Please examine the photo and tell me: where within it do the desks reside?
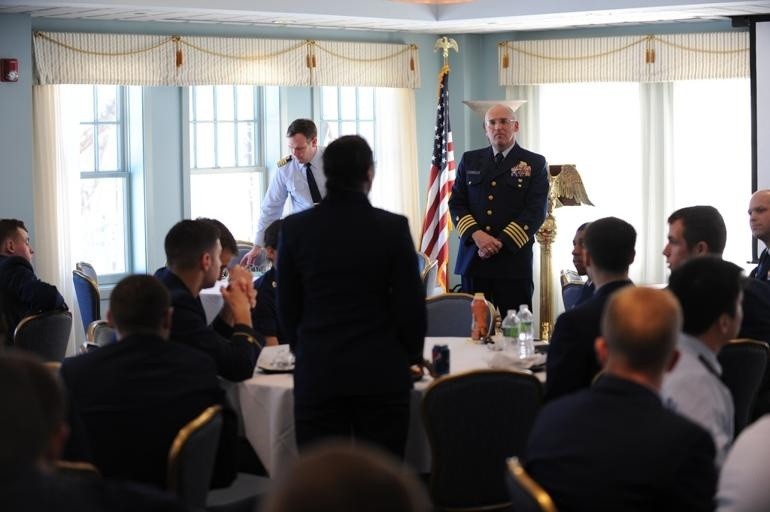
[238,336,554,474]
[193,271,261,324]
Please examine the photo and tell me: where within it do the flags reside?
[419,66,460,293]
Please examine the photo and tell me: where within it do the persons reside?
[448,103,551,314]
[0,189,770,512]
[239,119,328,271]
[273,134,426,460]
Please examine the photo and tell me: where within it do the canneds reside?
[432,344,449,378]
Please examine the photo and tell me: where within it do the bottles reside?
[471,292,535,362]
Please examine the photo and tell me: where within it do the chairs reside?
[500,450,556,512]
[422,369,542,510]
[425,292,495,338]
[98,404,229,511]
[71,264,116,347]
[14,311,72,365]
[715,335,768,433]
[561,282,588,312]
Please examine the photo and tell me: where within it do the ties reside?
[496,153,503,167]
[306,162,322,207]
